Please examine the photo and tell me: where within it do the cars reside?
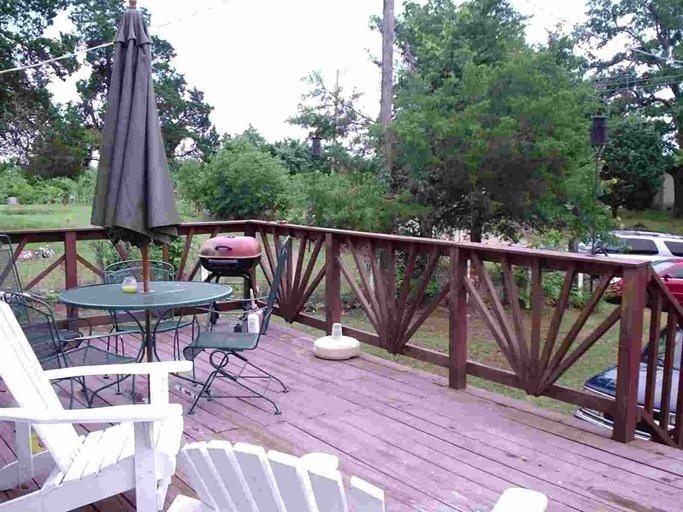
[574,325,681,444]
[566,229,681,312]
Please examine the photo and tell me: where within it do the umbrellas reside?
[89,2,180,292]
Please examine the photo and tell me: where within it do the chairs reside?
[165,437,549,512]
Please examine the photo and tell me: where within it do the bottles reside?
[121,275,138,293]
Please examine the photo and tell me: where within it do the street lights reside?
[309,128,321,222]
[588,105,609,297]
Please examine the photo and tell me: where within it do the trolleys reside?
[196,232,261,321]
